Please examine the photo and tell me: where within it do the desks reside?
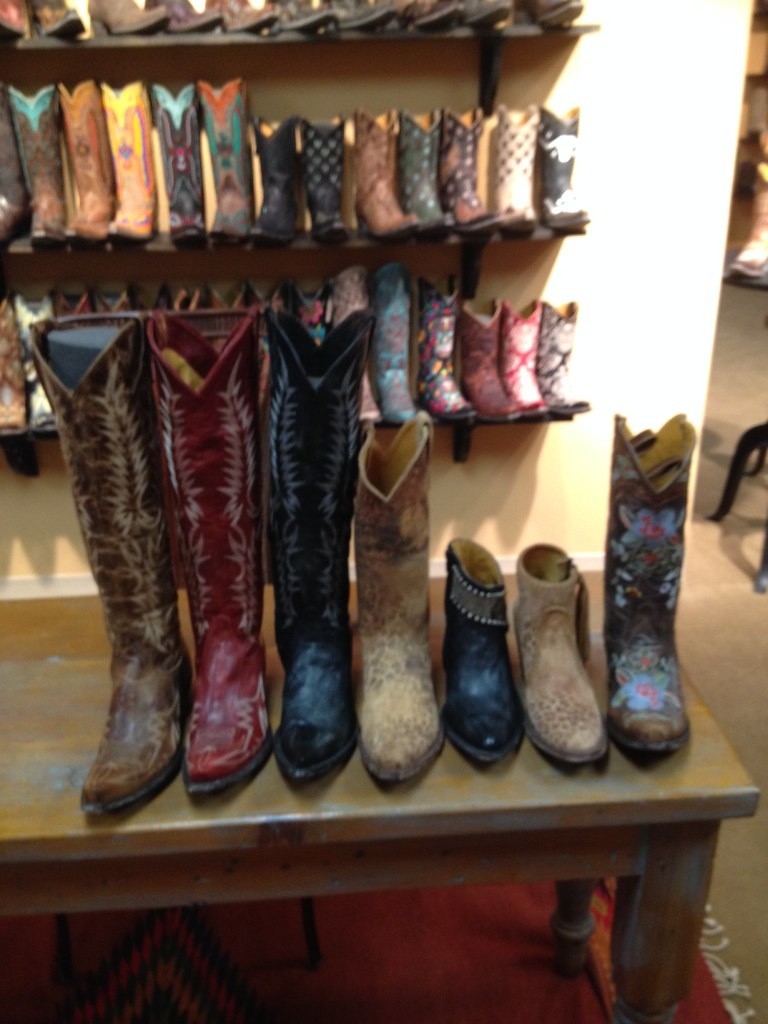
[0,574,760,1024]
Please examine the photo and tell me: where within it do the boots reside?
[0,262,590,440]
[440,538,523,762]
[9,84,68,246]
[0,82,28,245]
[604,414,697,754]
[150,83,206,245]
[0,0,584,45]
[354,107,419,238]
[495,104,537,232]
[266,305,358,790]
[249,116,295,243]
[57,80,112,244]
[352,412,441,784]
[398,108,456,234]
[197,78,254,241]
[442,108,502,233]
[100,81,158,243]
[540,104,590,228]
[146,307,271,796]
[28,311,191,816]
[299,116,347,236]
[515,544,606,763]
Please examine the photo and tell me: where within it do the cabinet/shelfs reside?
[0,0,601,479]
